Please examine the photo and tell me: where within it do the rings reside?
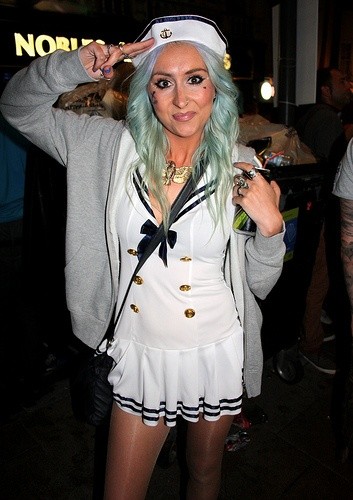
[108,44,115,56]
[236,174,249,189]
[237,186,243,195]
[120,43,124,54]
[245,165,258,180]
[123,53,128,59]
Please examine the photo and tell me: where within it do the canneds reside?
[263,151,293,166]
[232,166,270,236]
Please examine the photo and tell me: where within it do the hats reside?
[128,15,229,71]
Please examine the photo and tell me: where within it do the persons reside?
[0,14,286,500]
[294,66,353,374]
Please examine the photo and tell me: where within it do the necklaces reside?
[161,160,193,185]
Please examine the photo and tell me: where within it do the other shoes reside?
[324,332,335,342]
[320,310,331,325]
[304,345,337,376]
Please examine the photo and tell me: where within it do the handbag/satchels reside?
[88,354,114,425]
